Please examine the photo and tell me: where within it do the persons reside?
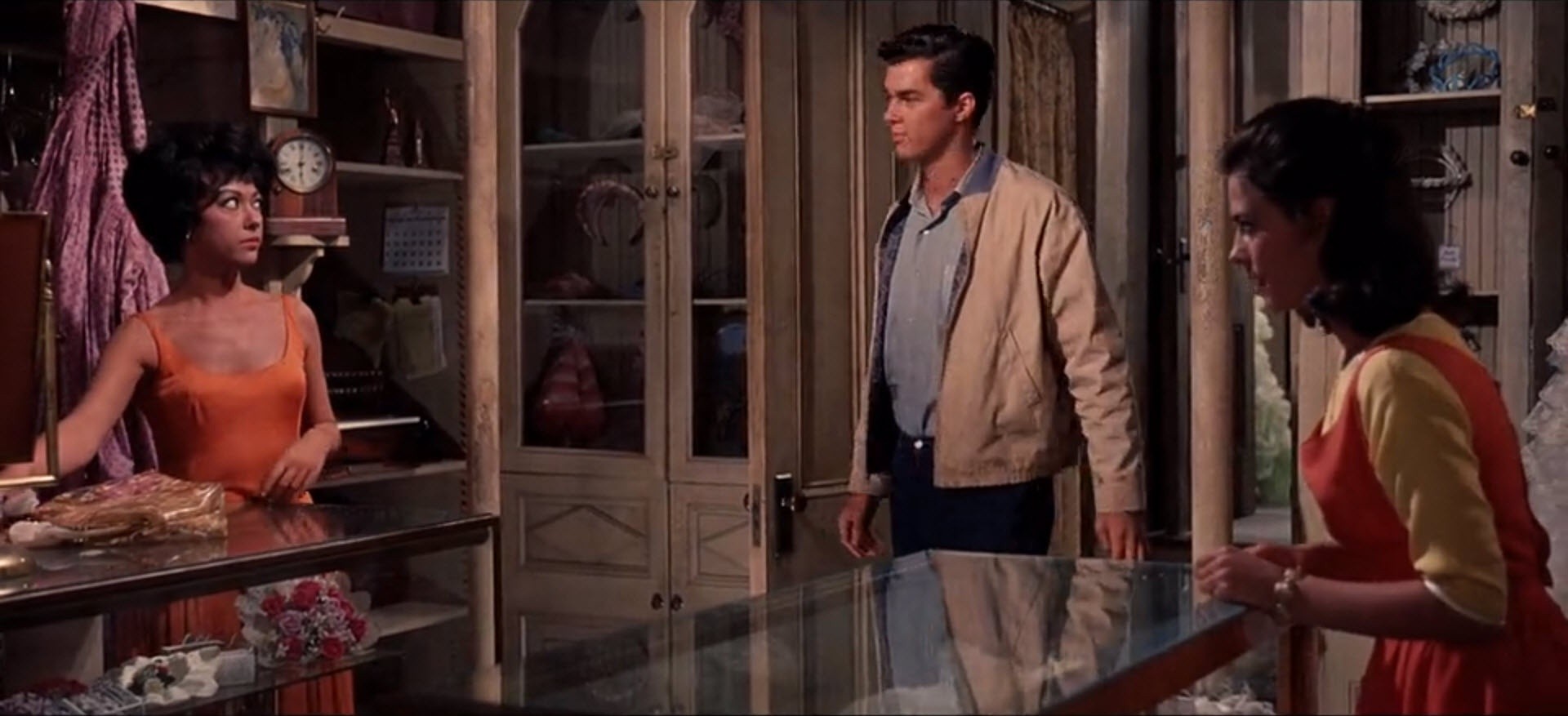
[1192,96,1568,716]
[837,21,1147,560]
[0,118,355,716]
[869,550,1134,716]
[522,214,606,447]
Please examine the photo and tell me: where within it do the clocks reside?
[263,127,347,238]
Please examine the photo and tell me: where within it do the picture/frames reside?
[242,0,319,119]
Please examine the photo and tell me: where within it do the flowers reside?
[233,571,374,666]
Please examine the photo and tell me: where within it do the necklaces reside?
[1328,367,1338,426]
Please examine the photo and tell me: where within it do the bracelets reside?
[1274,566,1309,615]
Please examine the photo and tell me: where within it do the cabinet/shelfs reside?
[309,13,466,641]
[1303,1,1568,716]
[497,0,800,662]
